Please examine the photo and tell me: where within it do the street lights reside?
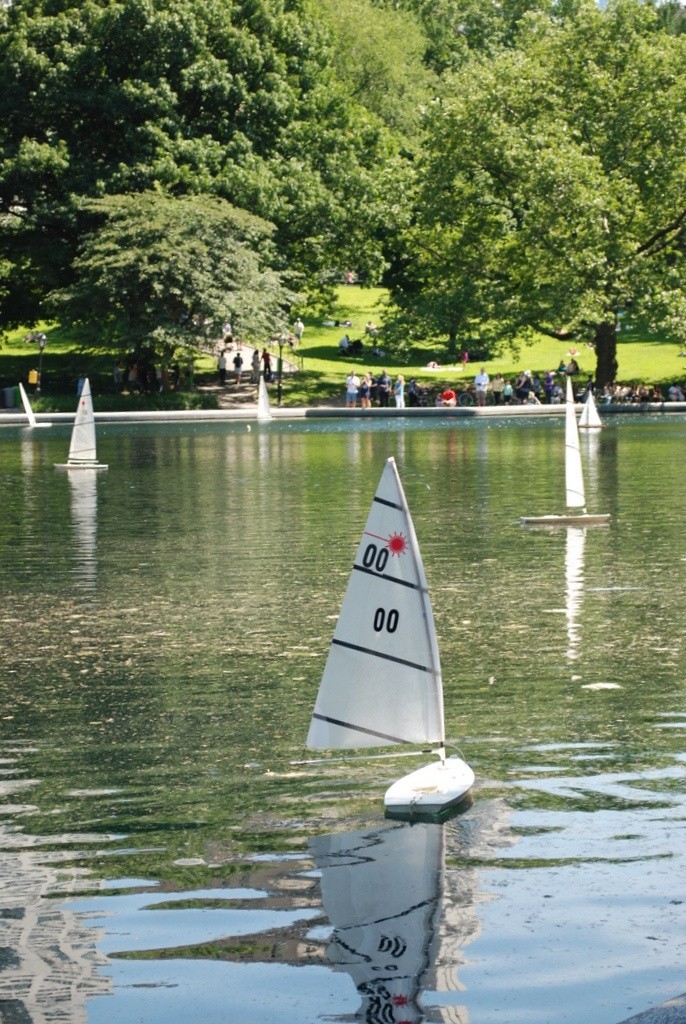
[276,334,287,407]
[33,334,49,402]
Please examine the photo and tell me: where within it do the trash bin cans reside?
[11,386,21,407]
[0,387,16,408]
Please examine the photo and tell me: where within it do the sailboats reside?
[253,375,277,421]
[18,382,54,429]
[303,453,477,822]
[576,389,608,429]
[518,374,614,526]
[304,813,471,1023]
[53,376,110,470]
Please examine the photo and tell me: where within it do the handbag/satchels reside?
[265,373,274,382]
[252,361,259,368]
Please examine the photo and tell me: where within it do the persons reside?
[23,270,686,407]
